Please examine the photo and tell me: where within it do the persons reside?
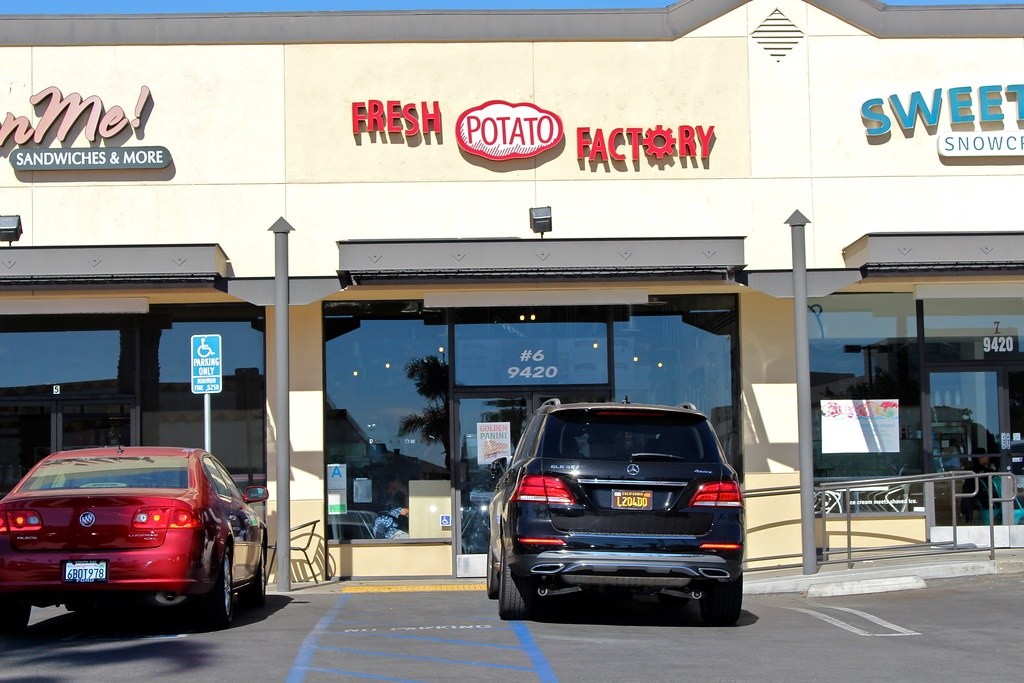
[372,471,408,539]
[960,447,1001,526]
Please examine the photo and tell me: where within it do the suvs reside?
[487,399,748,628]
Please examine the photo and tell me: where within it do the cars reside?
[0,447,269,639]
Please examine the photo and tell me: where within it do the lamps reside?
[529,205,552,238]
[0,214,23,246]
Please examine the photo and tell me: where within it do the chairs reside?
[972,477,1024,525]
[268,519,320,584]
[332,510,378,540]
